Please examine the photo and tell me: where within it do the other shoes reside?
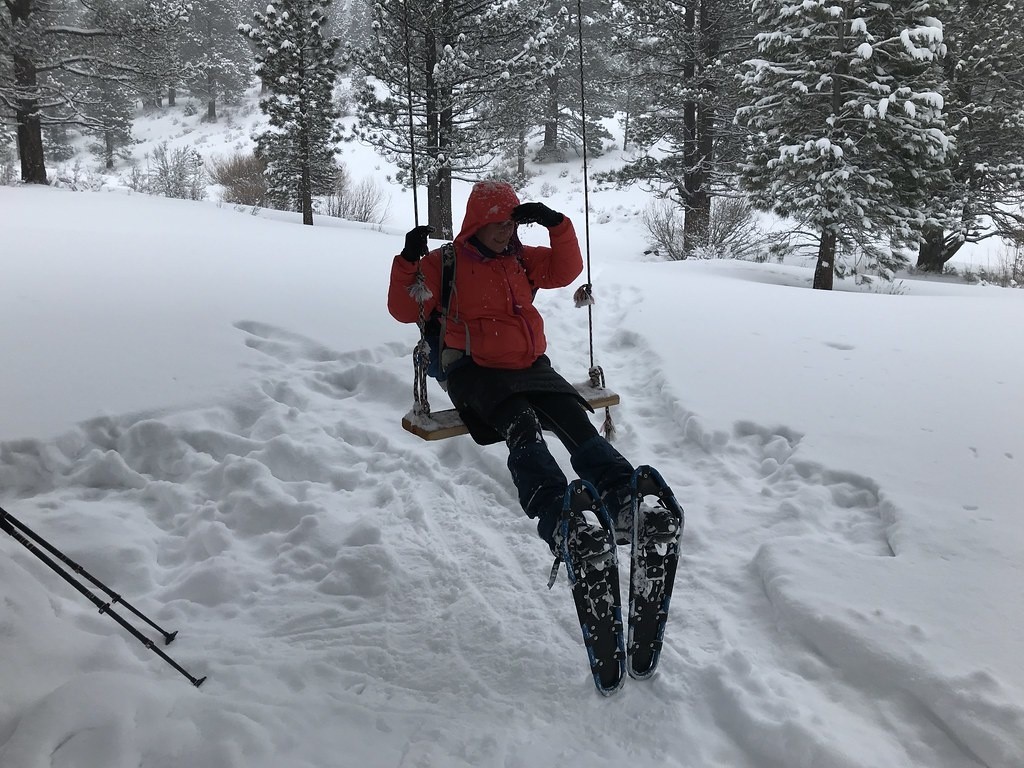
[615,495,678,545]
[551,522,608,561]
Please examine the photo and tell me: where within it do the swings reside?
[396,1,620,442]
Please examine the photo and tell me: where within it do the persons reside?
[385,178,672,567]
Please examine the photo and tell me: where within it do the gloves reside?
[400,225,437,265]
[511,202,563,228]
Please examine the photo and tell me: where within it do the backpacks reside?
[424,241,456,382]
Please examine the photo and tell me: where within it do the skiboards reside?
[561,463,681,695]
